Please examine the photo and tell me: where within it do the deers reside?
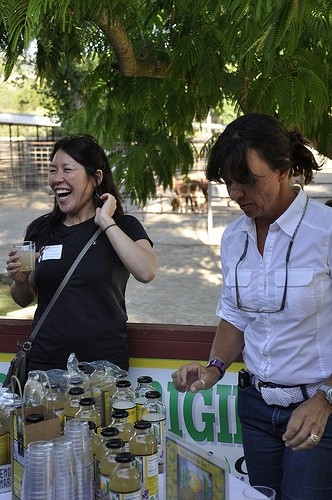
[171,178,209,214]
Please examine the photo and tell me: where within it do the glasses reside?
[63,133,99,153]
[235,240,293,314]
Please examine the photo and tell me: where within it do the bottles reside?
[23,370,43,409]
[0,388,22,466]
[42,383,65,436]
[25,414,44,425]
[65,363,167,500]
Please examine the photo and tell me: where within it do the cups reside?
[243,485,276,500]
[12,240,36,272]
[20,419,96,499]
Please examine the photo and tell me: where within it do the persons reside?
[6,133,156,396]
[172,112,332,500]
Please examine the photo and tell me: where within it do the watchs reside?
[317,384,332,405]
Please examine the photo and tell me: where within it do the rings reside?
[308,434,319,444]
[200,380,205,390]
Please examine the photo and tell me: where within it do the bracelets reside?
[205,358,225,381]
[104,224,117,233]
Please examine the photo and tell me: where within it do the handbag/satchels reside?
[2,351,27,397]
